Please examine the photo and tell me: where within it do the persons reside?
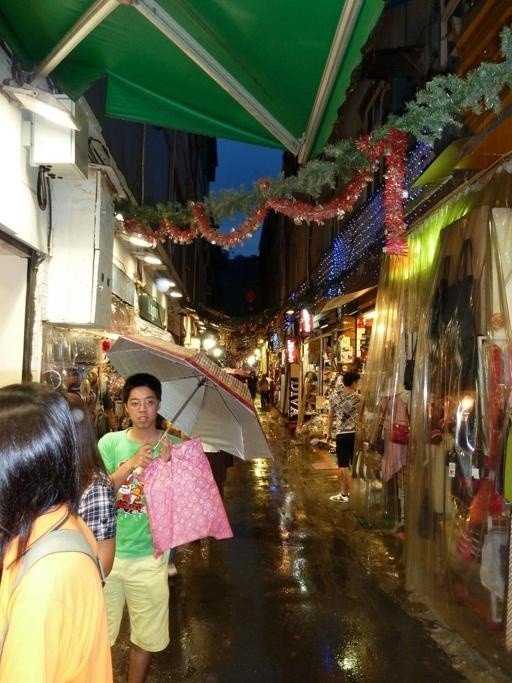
[0,382,114,683]
[249,371,273,412]
[96,373,188,683]
[325,371,362,503]
[62,392,116,578]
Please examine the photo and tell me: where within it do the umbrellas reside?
[107,334,274,465]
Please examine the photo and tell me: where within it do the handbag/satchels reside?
[480,529,509,600]
[403,358,415,390]
[445,275,474,325]
[366,434,386,456]
[133,436,233,559]
[390,424,411,445]
[352,451,382,481]
[354,428,369,450]
[429,286,443,339]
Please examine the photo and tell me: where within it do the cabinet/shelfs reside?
[300,316,355,447]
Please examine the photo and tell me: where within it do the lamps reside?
[130,251,162,264]
[115,230,152,248]
[342,305,359,315]
[2,84,82,132]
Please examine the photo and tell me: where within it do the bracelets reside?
[327,435,331,437]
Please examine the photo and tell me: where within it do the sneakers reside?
[168,561,177,576]
[329,492,350,502]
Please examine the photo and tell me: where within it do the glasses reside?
[127,399,161,409]
